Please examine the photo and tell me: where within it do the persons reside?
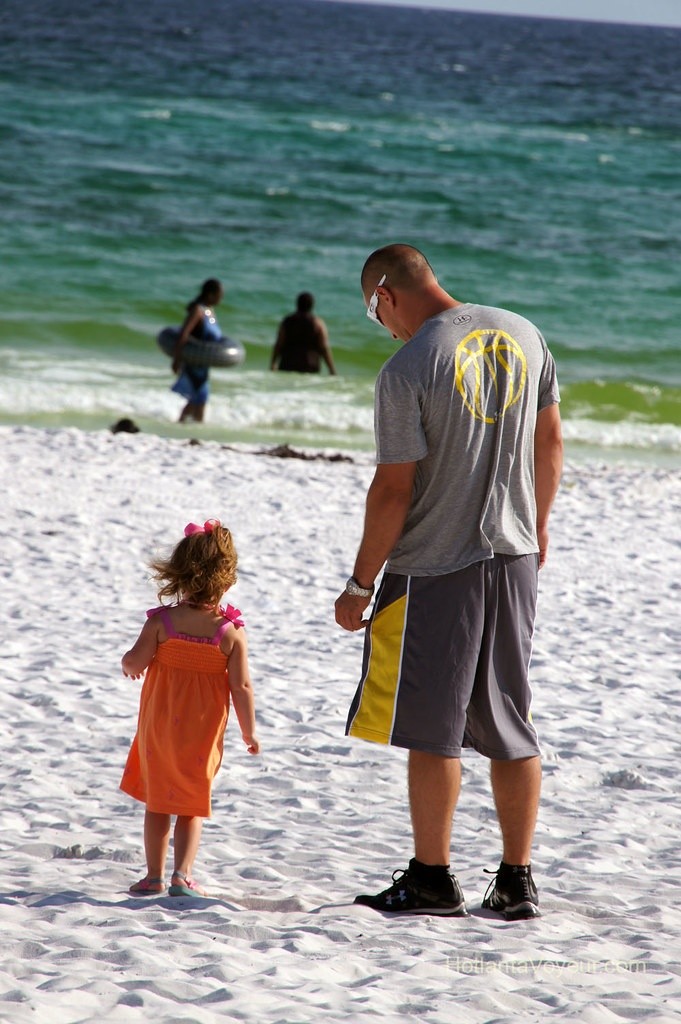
[172,277,226,425]
[334,244,565,922]
[268,291,336,376]
[120,520,260,897]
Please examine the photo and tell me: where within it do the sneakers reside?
[484,860,543,920]
[353,858,469,917]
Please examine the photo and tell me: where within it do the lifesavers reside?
[155,327,246,368]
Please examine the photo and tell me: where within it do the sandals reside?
[168,872,193,895]
[129,877,163,894]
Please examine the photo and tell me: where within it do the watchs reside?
[345,577,375,598]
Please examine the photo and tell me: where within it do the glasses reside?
[365,274,387,331]
